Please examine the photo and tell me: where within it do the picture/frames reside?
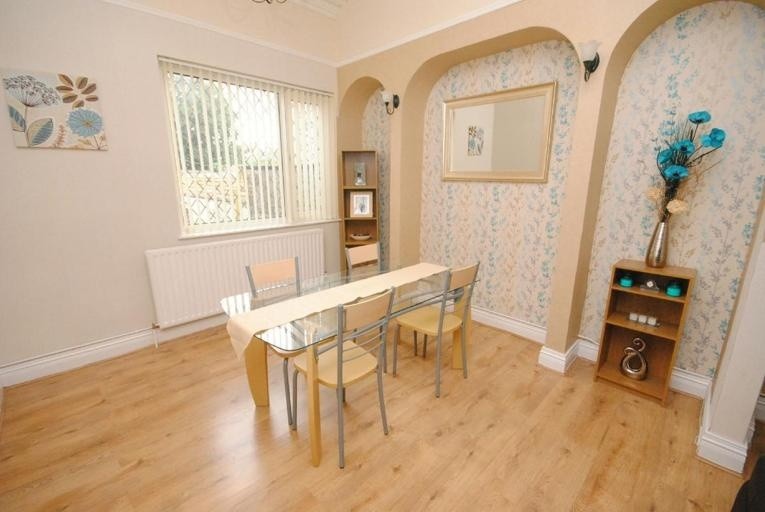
[348,189,375,221]
[437,81,559,185]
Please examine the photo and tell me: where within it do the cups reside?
[628,311,658,328]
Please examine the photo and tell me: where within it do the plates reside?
[349,234,372,240]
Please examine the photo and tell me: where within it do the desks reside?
[220,256,470,465]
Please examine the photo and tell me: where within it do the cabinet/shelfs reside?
[591,259,698,407]
[337,148,384,277]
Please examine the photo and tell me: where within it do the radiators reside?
[143,227,326,331]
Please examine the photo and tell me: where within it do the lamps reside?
[579,39,602,79]
[382,91,399,116]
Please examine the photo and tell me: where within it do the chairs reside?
[345,238,414,355]
[291,281,399,471]
[245,257,333,430]
[393,259,482,398]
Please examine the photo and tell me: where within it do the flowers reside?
[647,106,731,223]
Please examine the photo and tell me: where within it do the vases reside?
[647,216,668,267]
